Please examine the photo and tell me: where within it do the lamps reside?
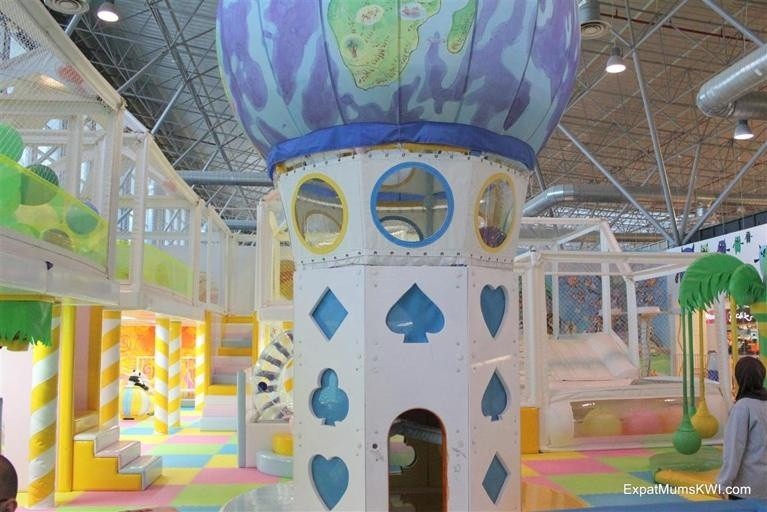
[604,47,627,74]
[96,0,119,22]
[733,119,754,140]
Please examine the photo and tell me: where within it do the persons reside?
[713,356,767,500]
[706,349,719,382]
[751,333,759,342]
[0,454,19,512]
[726,330,732,354]
[747,338,759,355]
[741,340,751,352]
